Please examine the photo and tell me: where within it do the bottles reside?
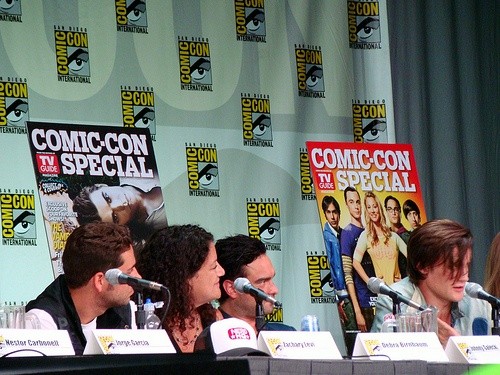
[145,299,162,329]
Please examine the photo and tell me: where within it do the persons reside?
[371,219,492,349]
[71,179,169,229]
[215,233,298,356]
[22,223,144,355]
[136,224,223,354]
[321,186,423,332]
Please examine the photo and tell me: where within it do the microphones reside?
[105,268,168,291]
[367,276,425,311]
[465,282,500,307]
[233,277,280,306]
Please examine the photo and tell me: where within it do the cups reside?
[301,315,320,332]
[396,308,438,336]
[3,306,40,329]
